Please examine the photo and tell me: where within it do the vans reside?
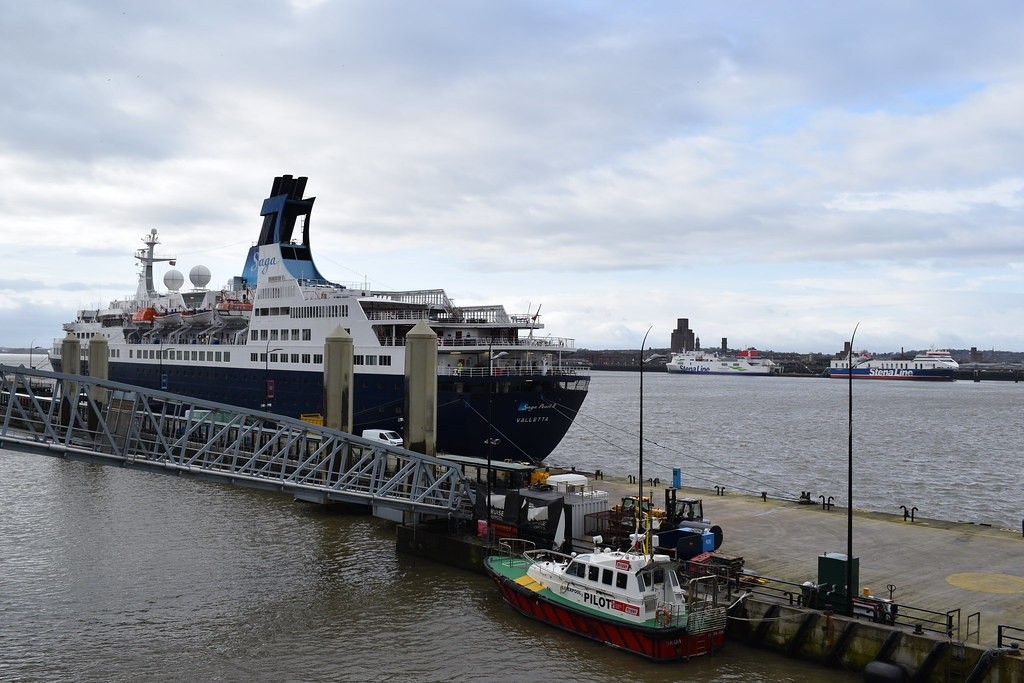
[361,429,404,448]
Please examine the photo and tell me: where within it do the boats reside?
[131,307,158,325]
[153,311,182,328]
[214,288,254,322]
[480,488,726,661]
[0,342,53,397]
[180,309,214,326]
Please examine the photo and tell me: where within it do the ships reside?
[46,174,592,467]
[827,347,960,382]
[666,346,785,375]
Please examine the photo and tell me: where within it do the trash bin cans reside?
[673,467,682,488]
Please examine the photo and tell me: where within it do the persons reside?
[505,361,510,369]
[515,361,521,375]
[532,362,537,369]
[457,360,463,372]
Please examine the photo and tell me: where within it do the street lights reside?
[639,323,668,533]
[484,344,508,543]
[260,332,283,412]
[847,322,878,620]
[159,338,176,391]
[29,339,42,389]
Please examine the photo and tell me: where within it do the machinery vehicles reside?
[612,492,667,520]
[659,486,704,532]
